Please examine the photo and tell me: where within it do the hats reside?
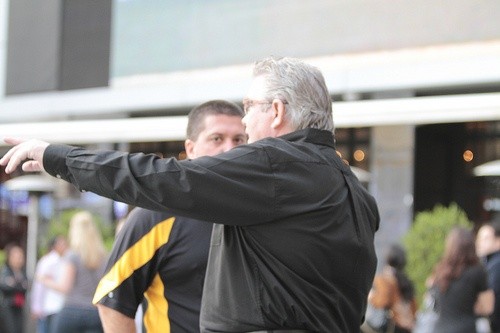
[448,226,471,245]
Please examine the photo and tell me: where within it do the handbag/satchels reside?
[367,311,386,331]
[412,299,438,333]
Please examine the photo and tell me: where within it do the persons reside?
[372,241,416,333]
[95,98,249,332]
[0,56,380,332]
[4,211,129,332]
[473,219,500,302]
[424,227,494,332]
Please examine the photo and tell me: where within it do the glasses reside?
[242,98,272,112]
